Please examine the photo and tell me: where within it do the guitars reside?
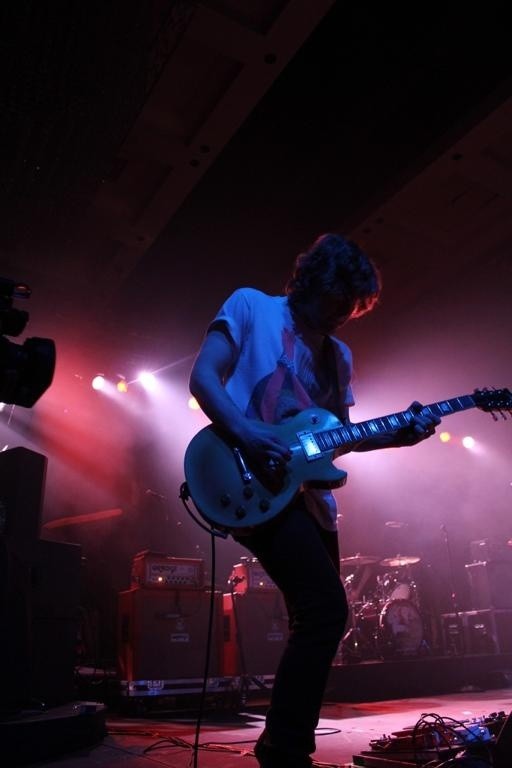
[183,388,512,535]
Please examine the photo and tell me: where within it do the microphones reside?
[440,525,448,535]
[145,489,168,501]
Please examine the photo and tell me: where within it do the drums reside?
[355,602,381,634]
[346,601,364,628]
[376,573,413,602]
[362,598,425,659]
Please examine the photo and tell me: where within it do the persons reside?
[189,234,442,767]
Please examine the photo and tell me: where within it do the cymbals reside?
[340,554,381,565]
[380,556,422,567]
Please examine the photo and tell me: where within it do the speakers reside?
[116,588,223,681]
[223,592,293,676]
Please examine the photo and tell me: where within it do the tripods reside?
[332,563,385,663]
